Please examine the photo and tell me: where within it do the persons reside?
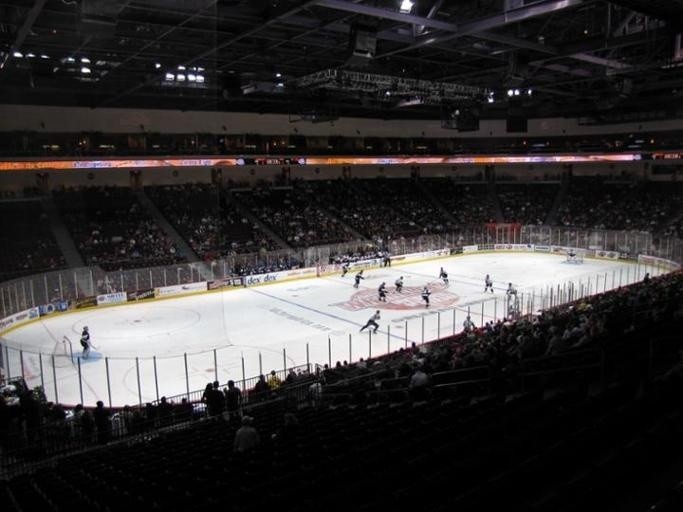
[334,307,560,364]
[352,268,365,288]
[0,365,681,510]
[247,174,559,278]
[482,273,494,294]
[376,281,388,303]
[393,275,404,293]
[560,174,682,365]
[505,282,518,298]
[0,181,248,318]
[436,266,449,288]
[79,325,93,359]
[420,285,431,307]
